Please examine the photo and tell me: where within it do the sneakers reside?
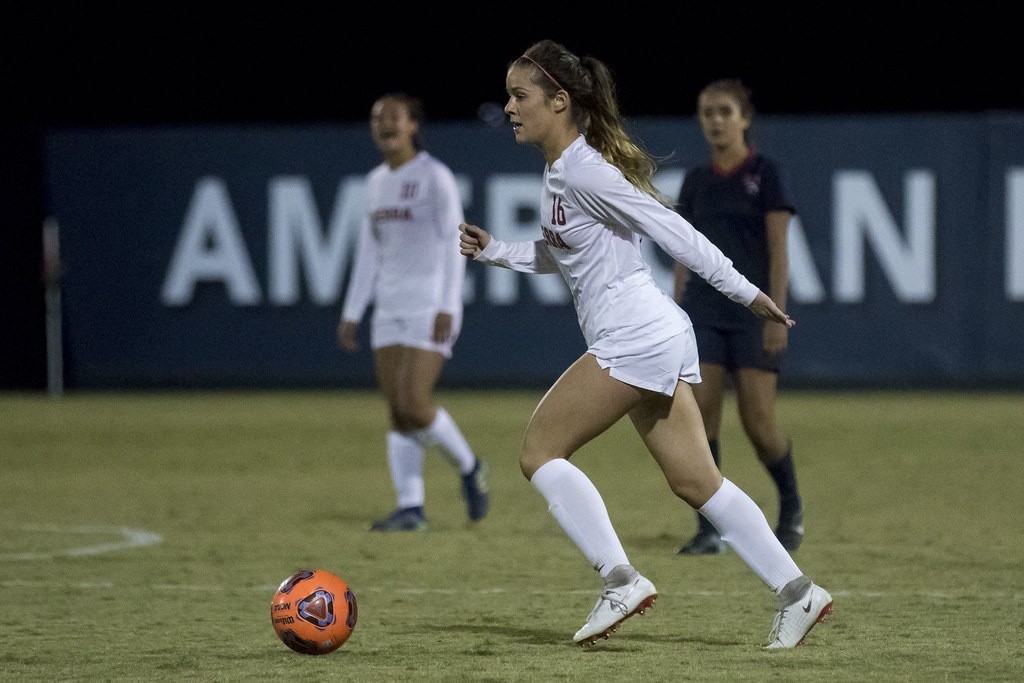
[573,573,658,647]
[775,501,806,550]
[370,506,429,533]
[763,580,834,649]
[460,455,491,521]
[678,526,722,555]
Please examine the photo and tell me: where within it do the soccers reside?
[269,568,359,656]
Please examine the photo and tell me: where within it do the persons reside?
[673,78,805,558]
[458,38,834,651]
[339,95,489,533]
